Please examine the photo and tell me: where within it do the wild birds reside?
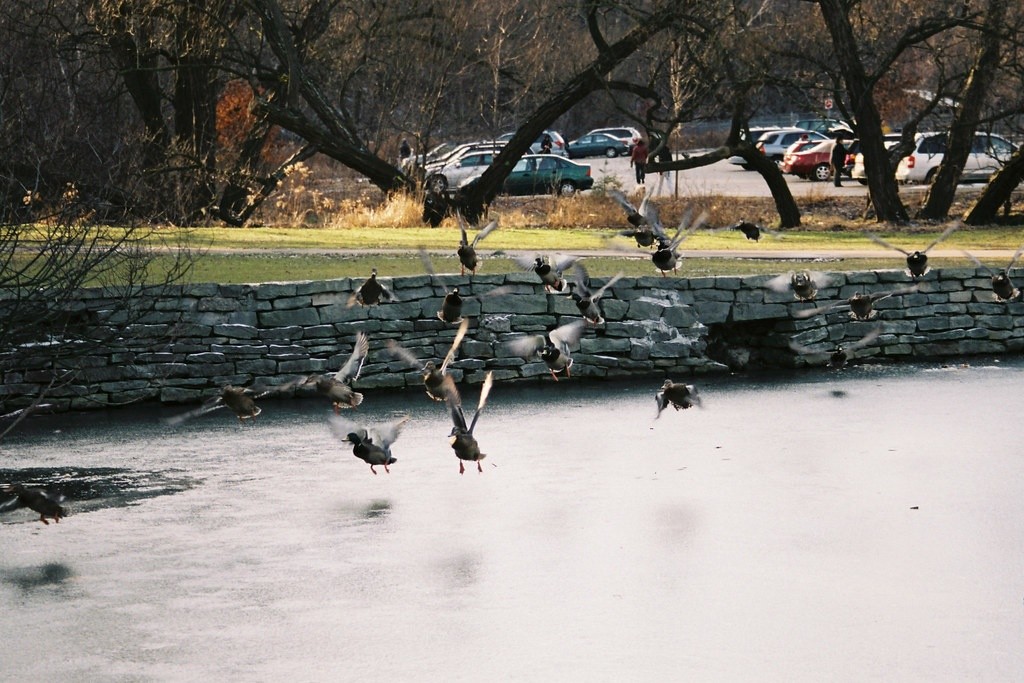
[0,480,73,525]
[963,247,1024,302]
[159,168,962,475]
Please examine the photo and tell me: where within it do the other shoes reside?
[835,185,844,187]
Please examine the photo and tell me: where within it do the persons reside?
[881,119,892,136]
[631,140,648,184]
[559,130,570,150]
[831,138,848,186]
[399,137,410,160]
[756,141,766,155]
[541,135,553,154]
[799,134,809,141]
[648,135,659,151]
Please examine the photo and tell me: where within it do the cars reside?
[498,153,594,198]
[893,129,1021,186]
[398,126,566,199]
[566,132,628,158]
[739,118,902,185]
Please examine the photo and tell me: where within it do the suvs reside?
[567,126,644,156]
